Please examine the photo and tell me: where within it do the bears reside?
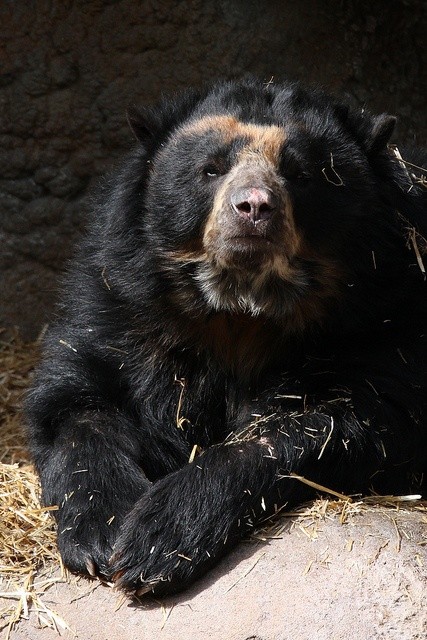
[21,72,427,600]
